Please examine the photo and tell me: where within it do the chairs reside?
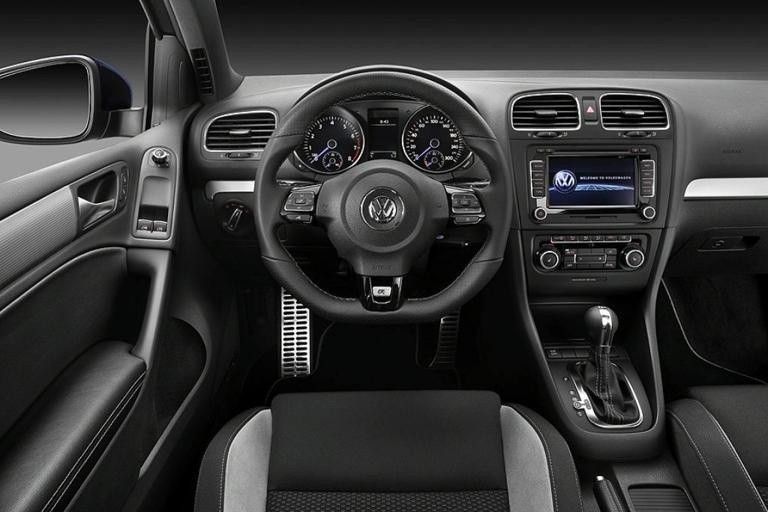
[668,385,767,511]
[191,384,581,511]
